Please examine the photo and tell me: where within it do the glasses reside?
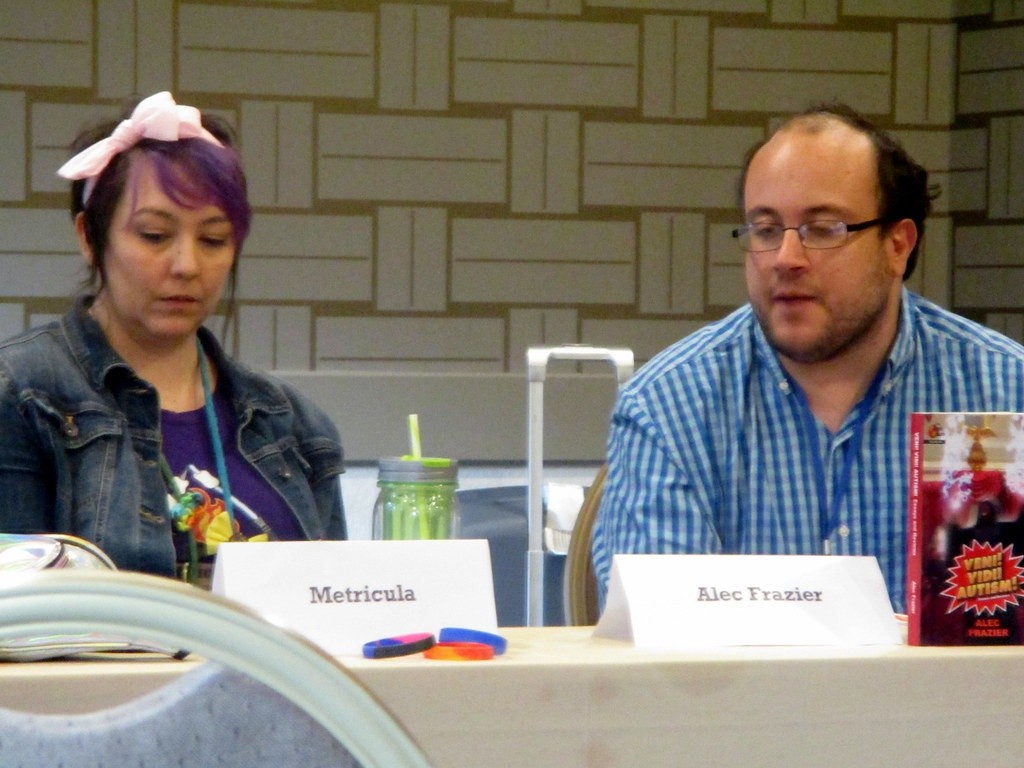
[732,218,893,253]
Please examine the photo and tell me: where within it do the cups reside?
[371,454,460,540]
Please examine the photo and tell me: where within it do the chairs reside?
[460,486,567,626]
[0,569,437,768]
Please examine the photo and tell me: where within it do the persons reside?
[592,102,1024,618]
[0,88,349,590]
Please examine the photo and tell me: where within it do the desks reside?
[0,625,1024,768]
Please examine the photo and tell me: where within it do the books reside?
[907,411,1024,647]
